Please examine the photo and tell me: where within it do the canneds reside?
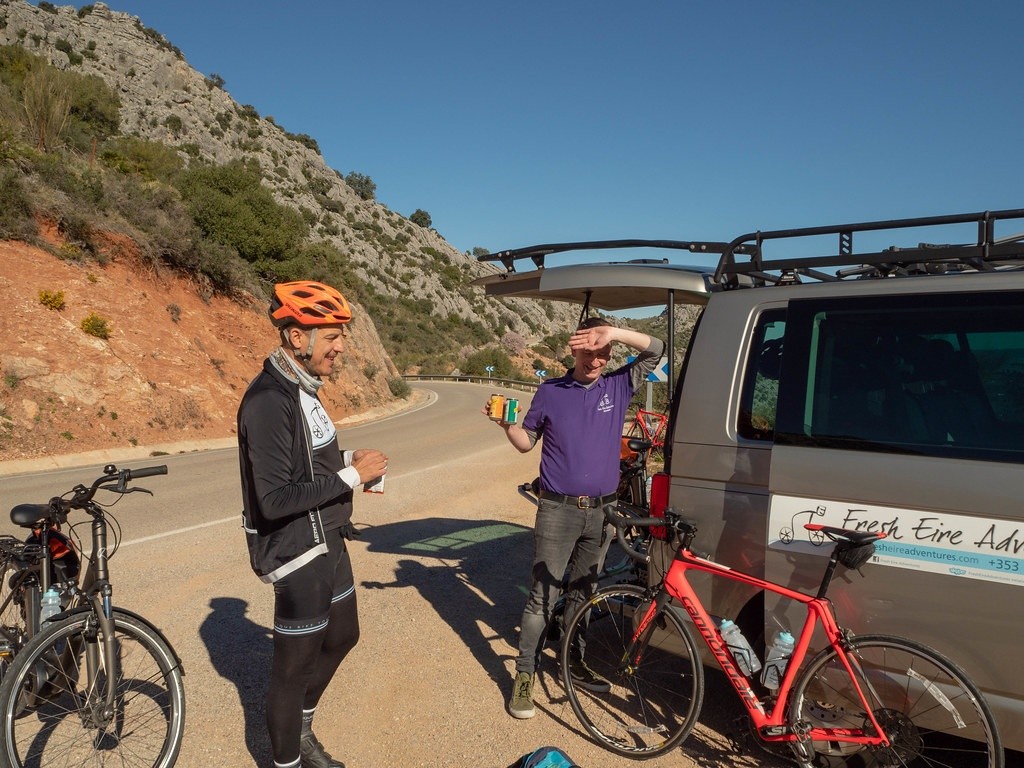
[489,393,504,420]
[504,397,518,425]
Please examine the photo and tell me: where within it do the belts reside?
[538,489,618,510]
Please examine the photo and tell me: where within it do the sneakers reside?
[508,671,536,718]
[558,660,612,692]
[300,733,345,768]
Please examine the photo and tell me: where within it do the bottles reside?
[760,629,795,689]
[645,476,652,503]
[39,588,61,631]
[719,618,762,676]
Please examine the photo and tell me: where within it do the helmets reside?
[268,280,352,328]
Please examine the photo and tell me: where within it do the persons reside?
[236,282,387,768]
[480,318,666,720]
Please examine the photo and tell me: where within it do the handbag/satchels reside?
[506,746,583,768]
[25,526,81,586]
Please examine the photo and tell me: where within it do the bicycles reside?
[561,500,1004,768]
[0,464,187,768]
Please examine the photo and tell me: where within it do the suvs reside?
[467,209,1024,755]
